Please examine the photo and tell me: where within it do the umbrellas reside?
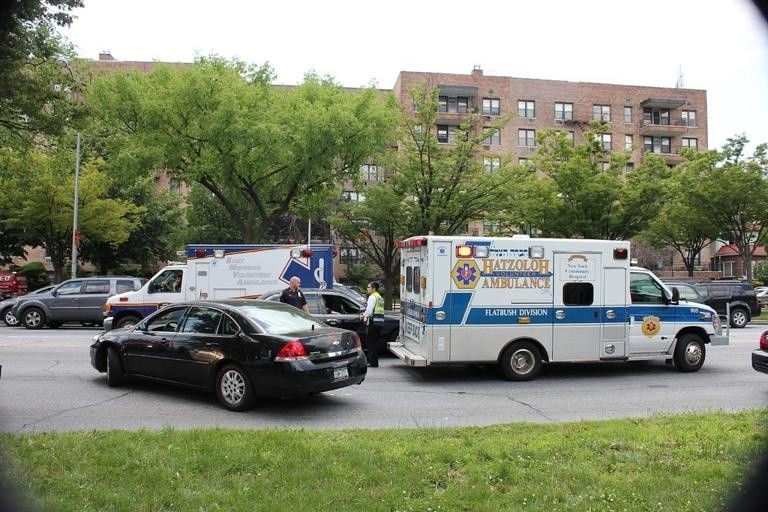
[366,363,379,367]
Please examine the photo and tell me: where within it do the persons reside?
[326,301,344,314]
[162,274,182,292]
[360,281,385,367]
[280,276,310,315]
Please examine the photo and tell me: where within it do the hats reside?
[635,275,768,375]
[258,287,402,357]
[333,282,367,306]
[0,285,96,330]
[86,298,370,414]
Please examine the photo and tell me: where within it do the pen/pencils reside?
[9,275,150,329]
